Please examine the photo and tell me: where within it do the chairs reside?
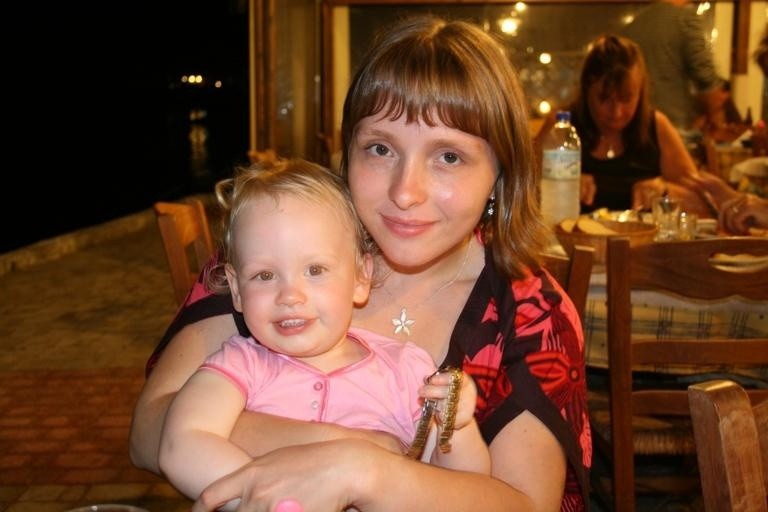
[153,199,216,304]
[516,123,768,512]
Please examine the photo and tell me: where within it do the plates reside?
[580,210,670,225]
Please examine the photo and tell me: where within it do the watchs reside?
[406,364,463,462]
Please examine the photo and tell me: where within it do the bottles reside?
[539,109,582,223]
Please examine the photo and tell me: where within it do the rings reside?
[729,206,739,216]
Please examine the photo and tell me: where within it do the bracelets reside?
[662,181,669,198]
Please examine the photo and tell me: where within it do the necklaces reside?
[370,227,473,336]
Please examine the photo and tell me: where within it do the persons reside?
[126,12,594,512]
[156,157,492,512]
[622,0,768,172]
[681,171,768,235]
[530,35,714,221]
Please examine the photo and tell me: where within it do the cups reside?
[652,196,682,240]
[679,212,699,240]
